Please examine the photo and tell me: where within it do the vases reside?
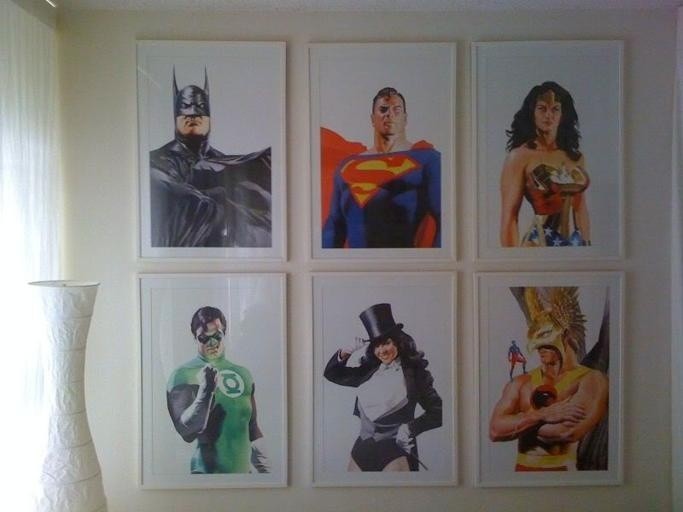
[27,275,113,511]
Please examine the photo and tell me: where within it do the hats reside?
[359,303,404,341]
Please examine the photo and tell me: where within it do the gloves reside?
[344,335,366,357]
[396,421,415,456]
[183,365,220,433]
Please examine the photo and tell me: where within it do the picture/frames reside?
[466,266,628,491]
[129,36,291,265]
[302,268,468,489]
[130,268,292,493]
[464,34,633,266]
[300,40,461,265]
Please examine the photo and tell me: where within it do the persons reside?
[166,305,275,473]
[494,80,593,247]
[488,310,609,472]
[321,299,442,472]
[149,61,275,251]
[505,340,529,381]
[321,87,442,249]
